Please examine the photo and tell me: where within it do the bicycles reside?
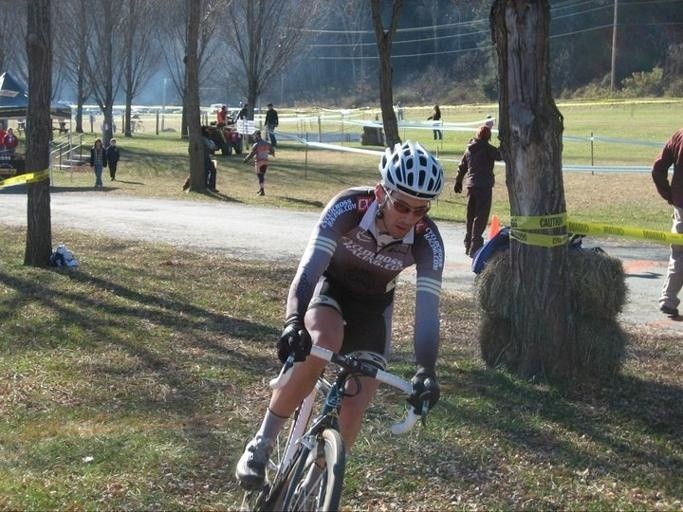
[130,120,145,133]
[238,342,429,512]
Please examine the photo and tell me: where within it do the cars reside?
[58,100,208,114]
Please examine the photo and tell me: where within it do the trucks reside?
[209,103,258,113]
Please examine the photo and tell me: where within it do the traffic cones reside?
[487,214,499,240]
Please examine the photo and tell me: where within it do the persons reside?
[2,128,18,152]
[397,101,404,121]
[236,140,445,512]
[485,116,494,141]
[131,112,141,133]
[90,140,107,188]
[427,105,442,140]
[201,103,278,196]
[652,129,683,314]
[106,138,120,181]
[0,123,6,152]
[454,126,504,258]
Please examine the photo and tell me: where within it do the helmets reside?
[378,139,443,201]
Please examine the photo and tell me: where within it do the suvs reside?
[201,119,245,155]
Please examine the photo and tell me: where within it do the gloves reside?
[277,313,312,363]
[454,182,462,193]
[406,359,440,415]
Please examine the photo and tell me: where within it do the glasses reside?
[380,185,431,216]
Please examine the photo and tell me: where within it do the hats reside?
[480,127,491,140]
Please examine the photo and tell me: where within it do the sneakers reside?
[661,306,678,314]
[235,436,273,489]
